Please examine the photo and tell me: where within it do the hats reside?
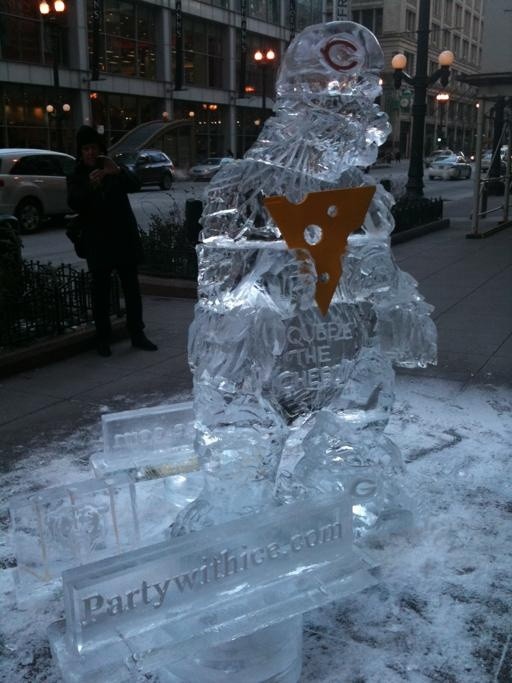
[74,123,110,162]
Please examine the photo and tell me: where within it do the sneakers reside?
[93,332,113,360]
[130,330,160,352]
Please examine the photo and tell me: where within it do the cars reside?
[426,141,512,183]
[188,156,236,182]
[432,90,450,148]
[0,146,84,230]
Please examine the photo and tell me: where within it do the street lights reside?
[254,45,278,137]
[390,52,455,201]
[39,0,71,150]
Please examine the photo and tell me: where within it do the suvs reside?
[107,149,179,194]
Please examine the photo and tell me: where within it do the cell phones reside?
[95,157,105,170]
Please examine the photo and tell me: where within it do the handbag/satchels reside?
[63,212,98,262]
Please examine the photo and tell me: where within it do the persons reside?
[385,151,392,163]
[64,124,158,358]
[211,151,218,164]
[459,148,466,160]
[380,149,385,161]
[226,150,233,157]
[395,151,401,164]
